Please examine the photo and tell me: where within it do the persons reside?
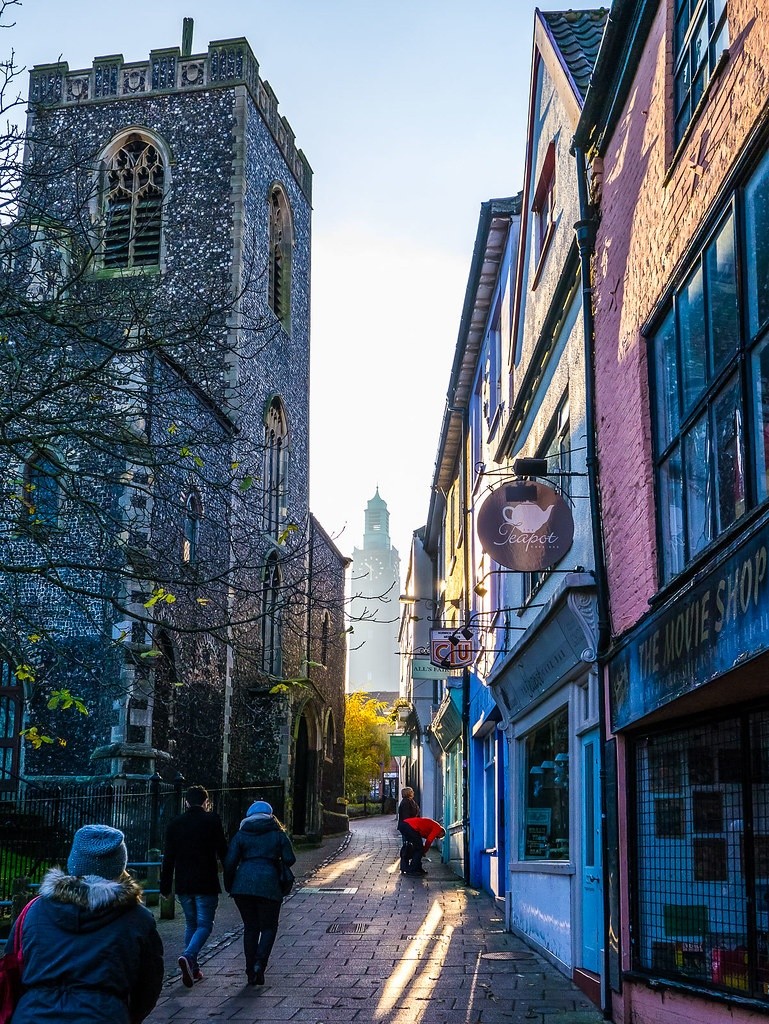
[397,787,421,876]
[225,801,297,987]
[398,817,446,875]
[160,787,228,988]
[0,825,165,1024]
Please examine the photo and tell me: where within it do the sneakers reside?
[178,956,204,988]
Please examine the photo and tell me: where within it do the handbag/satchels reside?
[400,842,414,860]
[1,895,45,1023]
[279,856,294,896]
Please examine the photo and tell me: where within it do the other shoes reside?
[411,862,428,875]
[245,964,266,986]
[401,865,415,874]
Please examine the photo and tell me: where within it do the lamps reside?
[449,625,526,646]
[473,569,575,598]
[530,751,569,797]
[510,458,548,477]
[462,603,544,641]
[440,649,509,667]
[399,595,459,609]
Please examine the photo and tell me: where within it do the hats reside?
[247,801,273,818]
[67,824,128,882]
